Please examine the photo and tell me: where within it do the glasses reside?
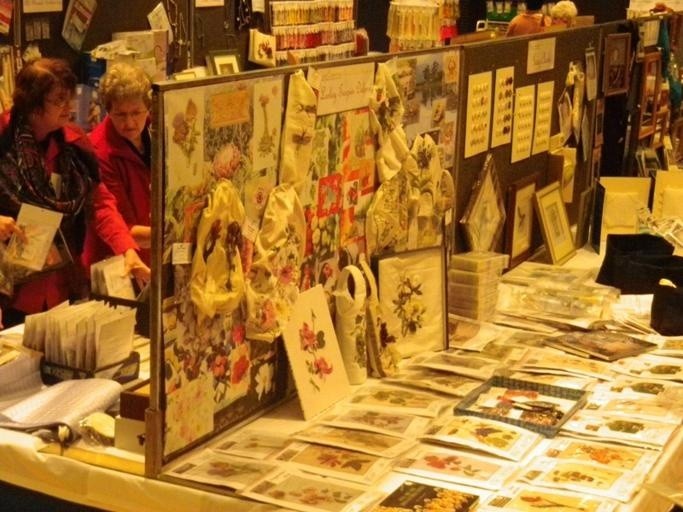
[112,108,149,120]
[46,97,66,108]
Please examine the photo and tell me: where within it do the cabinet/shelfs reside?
[636,47,658,154]
[655,106,673,151]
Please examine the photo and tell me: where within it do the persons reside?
[79,62,154,281]
[0,56,154,312]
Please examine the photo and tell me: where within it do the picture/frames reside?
[501,174,540,272]
[374,247,447,359]
[209,48,247,77]
[530,181,575,263]
[277,286,350,420]
[599,32,630,95]
[575,189,592,249]
[459,153,509,256]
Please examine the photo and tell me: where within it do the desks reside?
[1,14,681,512]
